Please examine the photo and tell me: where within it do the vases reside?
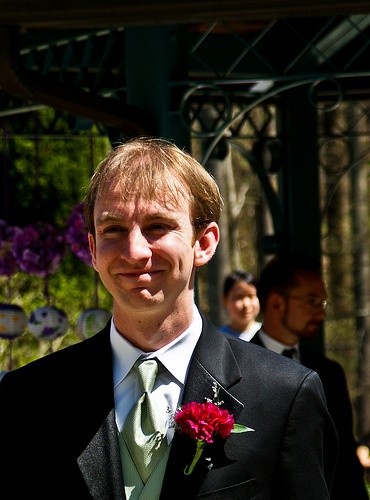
[78,307,112,341]
[0,301,26,339]
[29,303,69,341]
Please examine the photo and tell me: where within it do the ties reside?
[120,358,169,487]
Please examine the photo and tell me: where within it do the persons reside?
[0,137,370,500]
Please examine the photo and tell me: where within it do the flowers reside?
[69,203,95,268]
[164,380,256,476]
[10,225,65,278]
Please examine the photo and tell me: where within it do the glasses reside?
[285,294,328,312]
